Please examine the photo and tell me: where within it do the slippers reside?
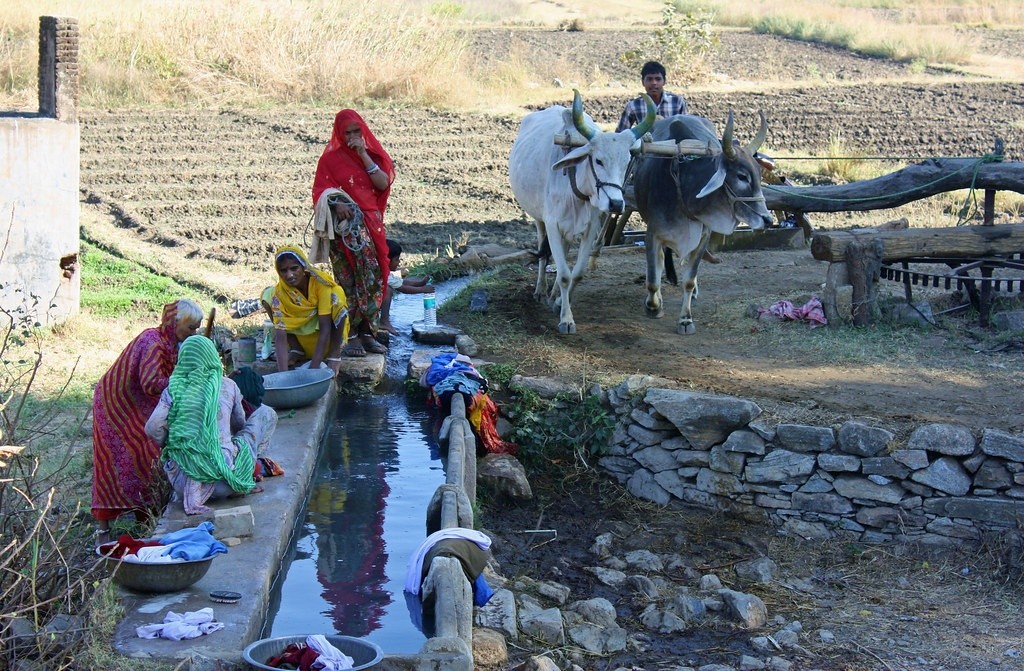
[344,343,366,356]
[362,341,388,353]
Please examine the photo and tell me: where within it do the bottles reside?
[423,278,436,327]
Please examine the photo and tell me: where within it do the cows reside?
[632,108,776,336]
[508,88,659,336]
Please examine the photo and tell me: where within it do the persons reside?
[91,240,434,544]
[313,109,396,356]
[615,62,726,265]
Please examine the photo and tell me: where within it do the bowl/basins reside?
[243,635,384,671]
[260,368,335,409]
[95,539,220,595]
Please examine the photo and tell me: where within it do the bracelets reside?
[366,164,381,176]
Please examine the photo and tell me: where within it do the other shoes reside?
[95,528,111,547]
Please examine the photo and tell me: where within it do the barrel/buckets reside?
[263,319,276,342]
[238,338,256,362]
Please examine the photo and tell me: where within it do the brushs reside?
[207,589,243,606]
